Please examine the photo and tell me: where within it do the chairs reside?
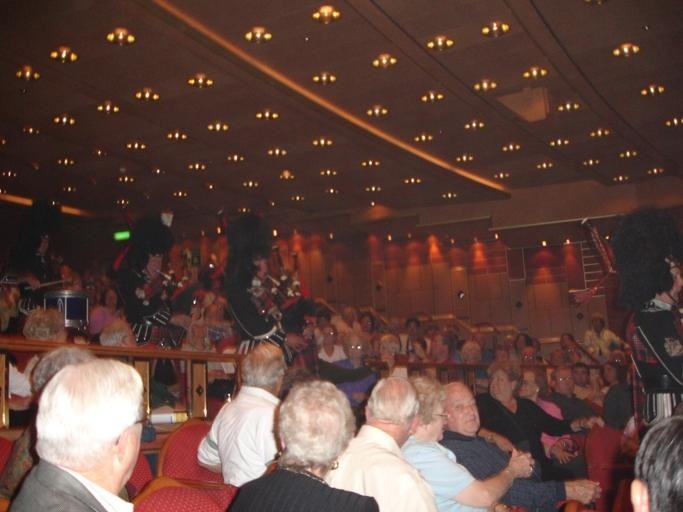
[0,418,237,511]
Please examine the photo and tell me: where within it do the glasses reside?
[559,376,573,382]
[326,330,338,337]
[451,402,477,412]
[116,411,149,445]
[524,349,623,364]
[350,344,364,352]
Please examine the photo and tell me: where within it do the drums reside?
[42,290,89,331]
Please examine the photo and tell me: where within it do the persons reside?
[0,208,683,512]
